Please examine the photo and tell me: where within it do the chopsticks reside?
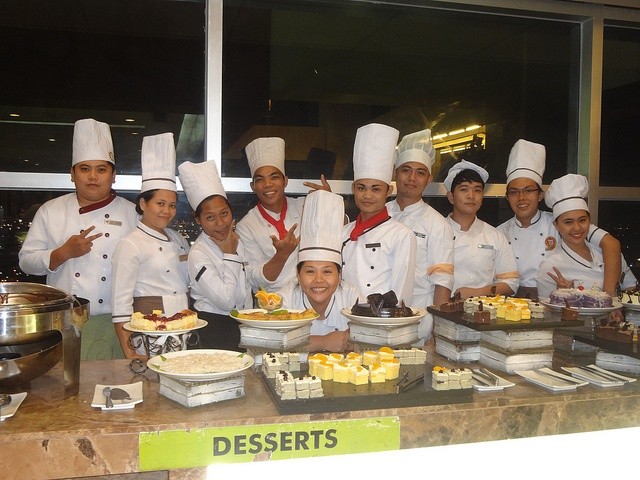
[574,363,629,384]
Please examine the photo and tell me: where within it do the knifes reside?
[103,387,111,408]
[532,366,579,385]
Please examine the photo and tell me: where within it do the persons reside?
[231,166,352,295]
[271,261,368,353]
[186,195,253,351]
[492,176,623,325]
[337,179,418,307]
[533,209,639,304]
[383,162,457,344]
[441,159,522,304]
[17,161,144,359]
[107,189,193,358]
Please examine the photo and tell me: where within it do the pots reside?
[0,281,90,389]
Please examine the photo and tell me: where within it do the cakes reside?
[461,293,546,325]
[548,288,614,308]
[592,317,640,344]
[351,289,413,318]
[391,346,430,365]
[262,351,301,379]
[306,346,400,386]
[273,368,324,401]
[130,309,198,334]
[429,366,475,391]
[620,288,640,305]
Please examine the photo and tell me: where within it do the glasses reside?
[505,189,541,196]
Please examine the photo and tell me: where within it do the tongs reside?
[395,371,425,395]
[468,365,499,386]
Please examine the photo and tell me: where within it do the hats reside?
[299,190,345,266]
[395,128,436,174]
[244,137,286,180]
[505,140,546,188]
[178,160,228,212]
[443,159,490,192]
[141,132,177,194]
[72,118,116,167]
[544,174,592,221]
[353,123,399,186]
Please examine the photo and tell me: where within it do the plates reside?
[537,295,623,316]
[340,306,428,325]
[123,318,208,336]
[620,302,640,312]
[146,349,254,382]
[470,367,516,391]
[229,309,320,330]
[91,380,144,411]
[560,364,637,388]
[0,392,27,422]
[513,367,590,392]
